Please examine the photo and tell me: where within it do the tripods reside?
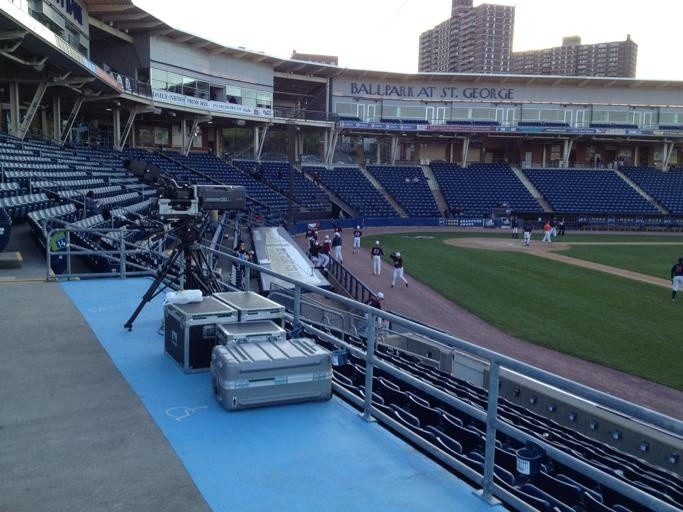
[124,242,224,331]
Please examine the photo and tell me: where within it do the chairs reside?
[1,136,682,292]
[264,308,682,510]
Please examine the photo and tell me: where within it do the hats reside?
[334,232,340,235]
[395,252,400,257]
[357,225,360,229]
[376,240,379,245]
[377,292,384,300]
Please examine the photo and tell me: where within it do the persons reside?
[304,220,343,273]
[370,240,384,276]
[352,224,361,254]
[670,257,683,302]
[230,239,251,271]
[84,189,110,220]
[389,252,408,288]
[208,209,218,236]
[509,214,565,248]
